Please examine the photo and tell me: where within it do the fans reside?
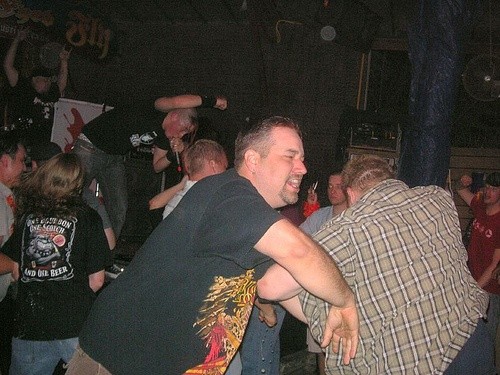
[462,54,500,102]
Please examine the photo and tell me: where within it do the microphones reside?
[176,152,181,172]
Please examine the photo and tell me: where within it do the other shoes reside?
[104,264,123,280]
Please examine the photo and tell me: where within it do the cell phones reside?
[311,181,319,194]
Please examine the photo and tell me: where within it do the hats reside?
[30,67,57,76]
[29,142,61,160]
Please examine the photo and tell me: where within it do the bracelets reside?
[196,95,217,108]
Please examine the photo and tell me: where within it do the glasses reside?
[484,183,500,190]
[327,182,343,189]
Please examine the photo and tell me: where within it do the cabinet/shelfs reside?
[337,148,402,181]
[116,160,183,259]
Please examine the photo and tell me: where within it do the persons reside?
[0,95,500,375]
[4,31,73,164]
